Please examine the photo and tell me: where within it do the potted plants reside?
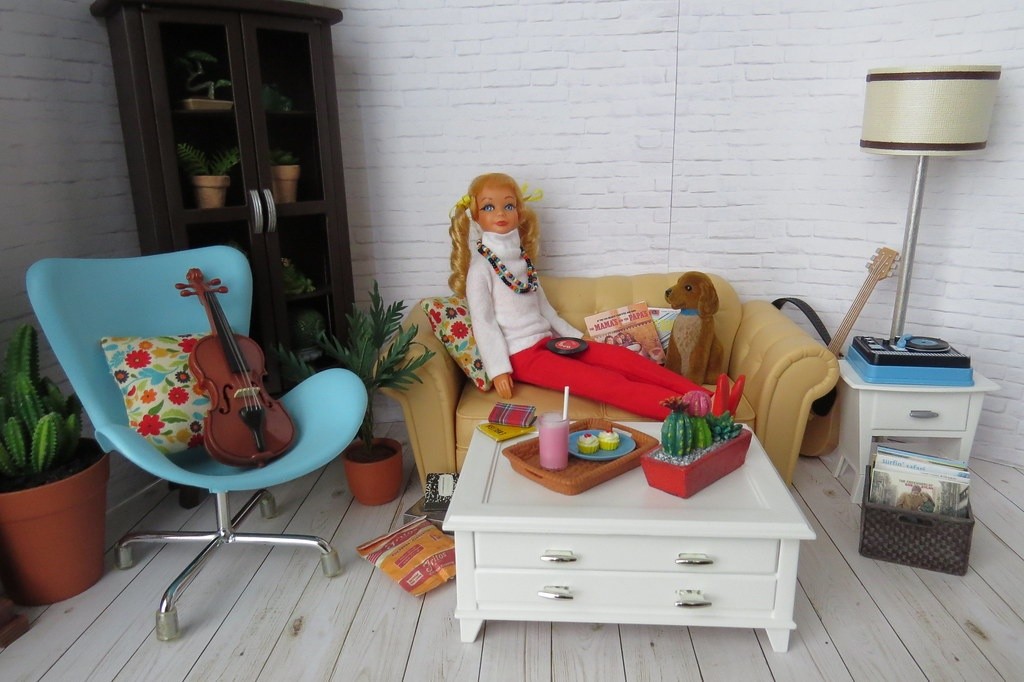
[291,308,322,359]
[338,281,405,504]
[1,328,109,604]
[640,391,751,498]
[183,49,300,206]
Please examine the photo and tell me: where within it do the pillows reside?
[421,292,492,389]
[100,334,209,452]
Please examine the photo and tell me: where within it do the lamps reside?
[857,65,1002,338]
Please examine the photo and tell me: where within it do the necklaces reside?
[472,238,539,295]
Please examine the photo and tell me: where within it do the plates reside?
[568,430,636,461]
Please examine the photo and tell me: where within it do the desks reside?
[836,361,1001,500]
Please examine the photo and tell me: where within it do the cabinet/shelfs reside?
[90,0,354,363]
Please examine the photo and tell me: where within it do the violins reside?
[175,268,296,468]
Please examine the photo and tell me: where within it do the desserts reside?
[577,428,620,454]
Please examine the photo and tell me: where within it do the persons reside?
[446,174,745,423]
[604,332,642,352]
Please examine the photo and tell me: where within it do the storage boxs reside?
[859,464,974,575]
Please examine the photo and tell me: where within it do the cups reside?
[538,411,570,469]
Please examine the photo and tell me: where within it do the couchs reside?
[379,274,839,489]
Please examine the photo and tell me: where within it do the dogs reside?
[664,271,724,386]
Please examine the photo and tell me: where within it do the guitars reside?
[799,247,901,457]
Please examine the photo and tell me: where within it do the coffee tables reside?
[442,422,817,653]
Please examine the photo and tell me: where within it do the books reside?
[869,445,971,517]
[585,299,668,367]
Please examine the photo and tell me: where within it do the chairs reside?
[26,246,368,639]
